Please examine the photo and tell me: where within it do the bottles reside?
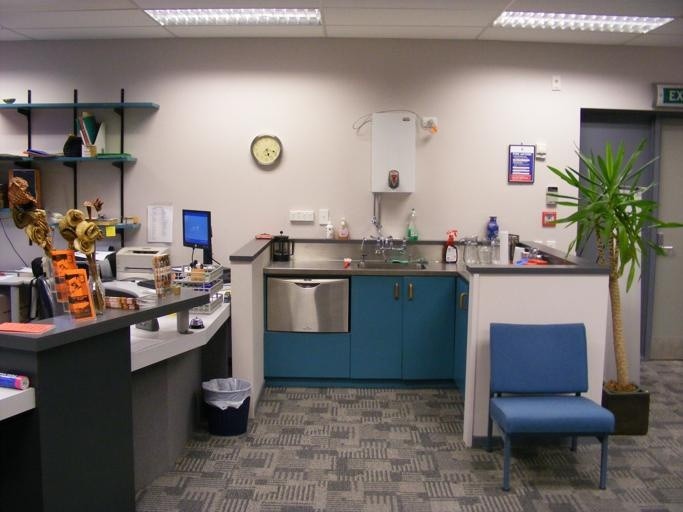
[485,216,499,239]
[272,231,294,262]
[455,238,499,266]
[326,217,335,239]
[508,233,543,265]
[407,208,418,241]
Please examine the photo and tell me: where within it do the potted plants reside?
[546,136,683,436]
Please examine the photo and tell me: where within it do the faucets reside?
[375,235,395,263]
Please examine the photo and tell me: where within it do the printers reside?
[116,246,169,281]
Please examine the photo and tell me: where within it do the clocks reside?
[250,134,283,167]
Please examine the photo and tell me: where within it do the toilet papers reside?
[499,229,509,265]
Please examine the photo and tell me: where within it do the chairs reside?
[487,322,616,491]
[31,256,91,318]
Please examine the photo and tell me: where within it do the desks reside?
[130,282,232,492]
[0,387,36,422]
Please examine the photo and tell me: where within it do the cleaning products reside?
[442,228,458,263]
[406,207,419,242]
[327,221,335,239]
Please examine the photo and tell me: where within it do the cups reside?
[343,257,352,269]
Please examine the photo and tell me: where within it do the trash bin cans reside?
[201,376,252,437]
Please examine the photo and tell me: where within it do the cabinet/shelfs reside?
[351,274,457,389]
[0,88,160,249]
[455,277,470,399]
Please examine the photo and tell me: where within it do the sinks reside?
[356,256,428,270]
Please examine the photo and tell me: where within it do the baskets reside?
[170,264,223,315]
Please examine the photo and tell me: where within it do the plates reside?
[2,98,16,104]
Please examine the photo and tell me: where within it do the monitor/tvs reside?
[182,209,212,250]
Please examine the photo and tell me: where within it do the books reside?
[0,322,56,335]
